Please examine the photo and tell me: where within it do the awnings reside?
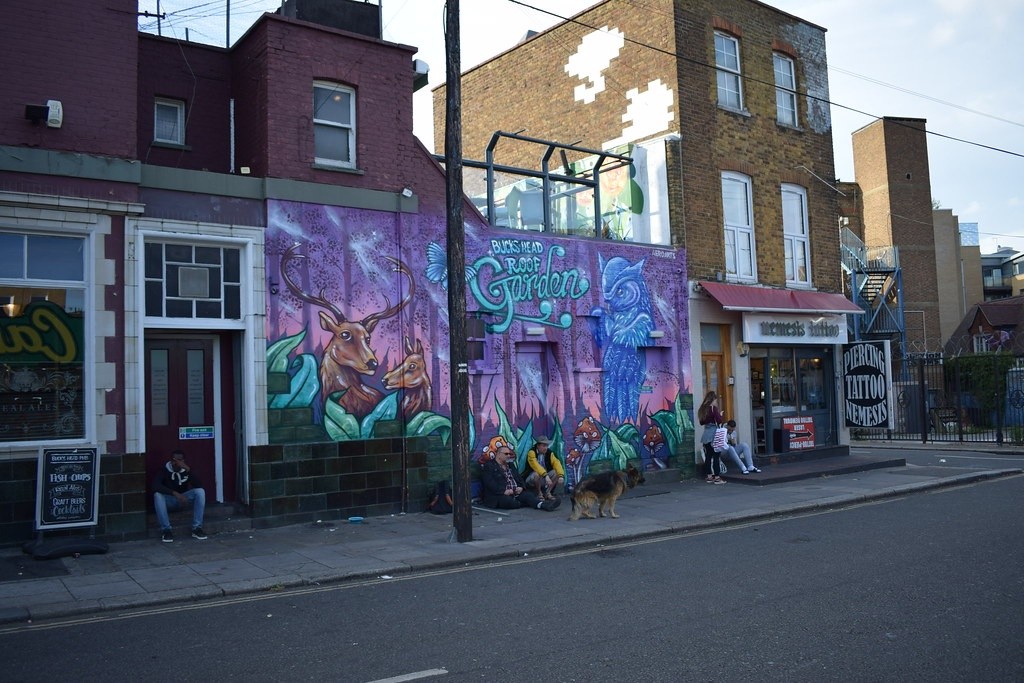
[694,281,867,314]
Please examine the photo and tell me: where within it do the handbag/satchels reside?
[712,426,729,452]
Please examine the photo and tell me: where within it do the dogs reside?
[566,463,645,521]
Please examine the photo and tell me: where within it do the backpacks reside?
[427,480,453,514]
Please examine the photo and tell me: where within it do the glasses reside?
[500,452,511,455]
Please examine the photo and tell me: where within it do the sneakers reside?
[162,531,174,542]
[192,529,208,540]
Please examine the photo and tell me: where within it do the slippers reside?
[747,467,762,473]
[741,469,749,475]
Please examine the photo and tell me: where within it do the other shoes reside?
[707,476,715,483]
[544,491,555,500]
[541,498,561,511]
[536,492,545,501]
[713,477,727,484]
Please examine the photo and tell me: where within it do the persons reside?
[153,452,208,541]
[480,447,562,511]
[720,419,762,475]
[522,435,564,501]
[698,390,727,484]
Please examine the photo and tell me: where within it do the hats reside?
[534,436,553,444]
